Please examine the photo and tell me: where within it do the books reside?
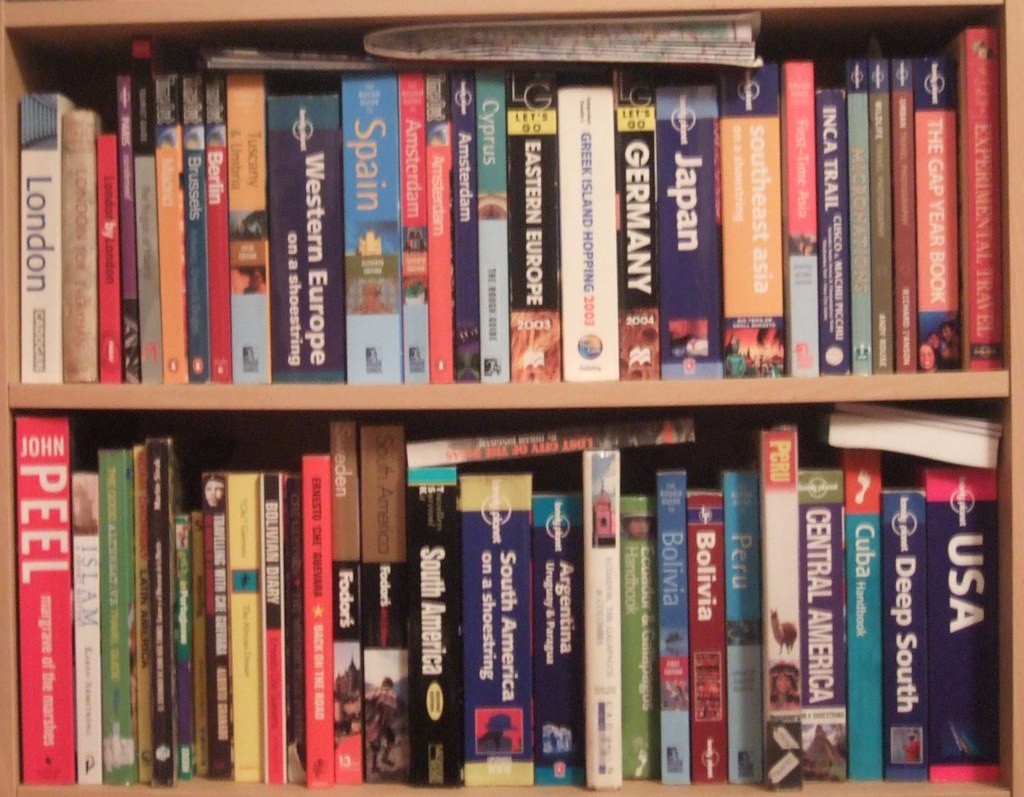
[12,17,1010,789]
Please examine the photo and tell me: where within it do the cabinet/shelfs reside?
[0,0,1024,797]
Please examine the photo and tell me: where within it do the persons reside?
[926,330,949,358]
[369,678,397,772]
[203,473,225,514]
[770,662,802,706]
[904,736,921,761]
[918,342,936,371]
[939,320,960,350]
